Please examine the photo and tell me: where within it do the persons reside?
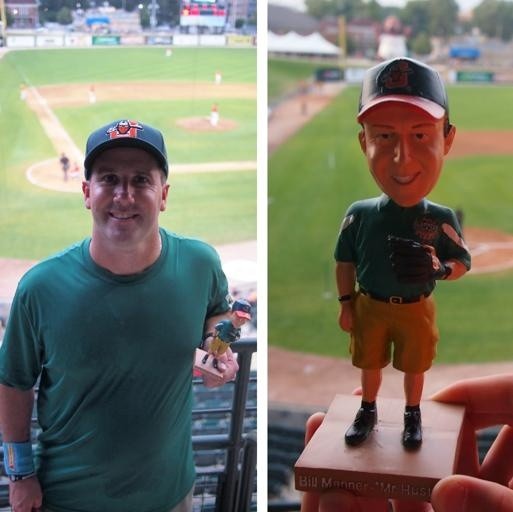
[201,298,252,368]
[269,73,323,151]
[0,119,241,512]
[299,370,512,512]
[11,41,228,187]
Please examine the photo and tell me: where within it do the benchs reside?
[268,395,510,512]
[0,369,256,512]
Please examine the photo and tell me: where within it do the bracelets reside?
[1,440,33,476]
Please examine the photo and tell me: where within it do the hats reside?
[84,119,169,178]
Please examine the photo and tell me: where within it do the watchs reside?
[6,474,37,483]
